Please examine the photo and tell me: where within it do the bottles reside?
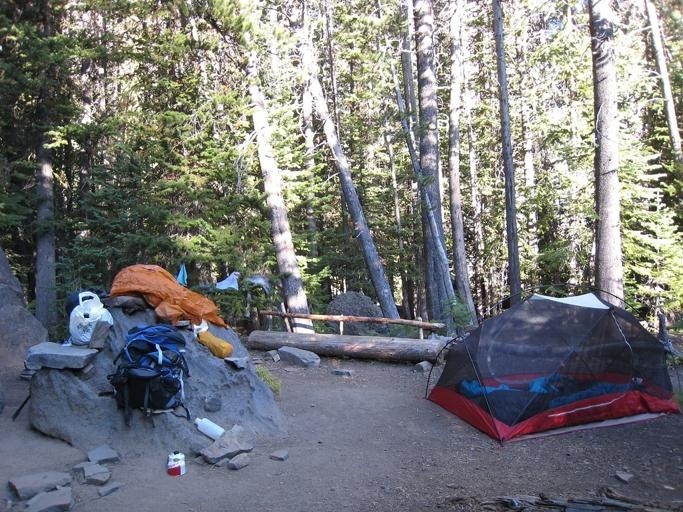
[193,417,224,440]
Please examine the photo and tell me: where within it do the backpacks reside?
[111,325,189,410]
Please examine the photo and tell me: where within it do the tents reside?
[424,284,683,448]
